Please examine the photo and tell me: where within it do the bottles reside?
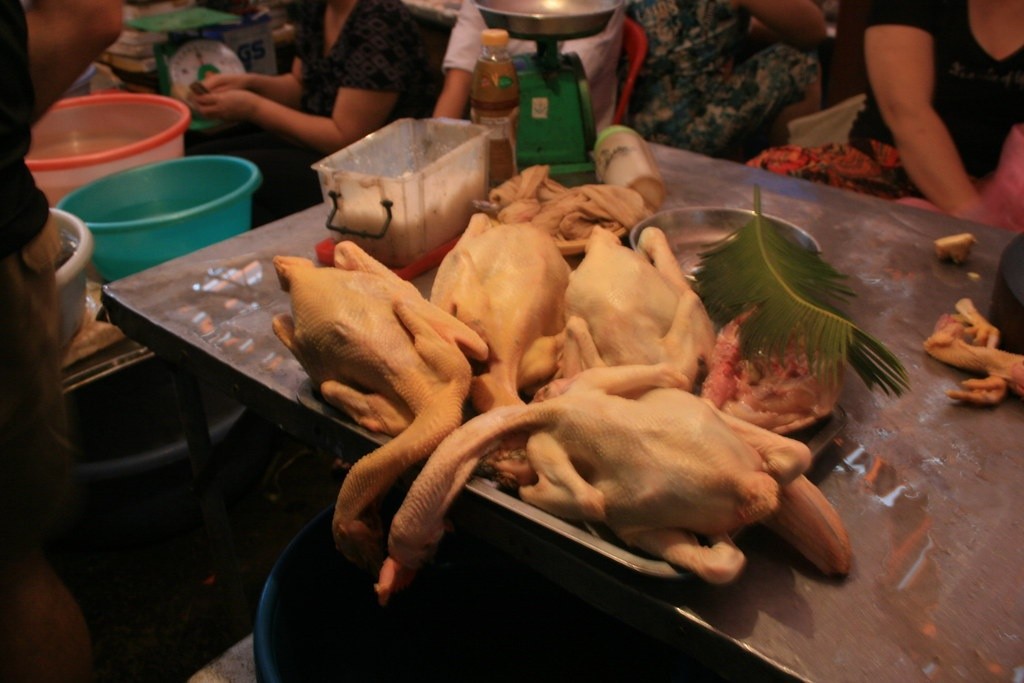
[468,28,520,189]
[592,118,666,213]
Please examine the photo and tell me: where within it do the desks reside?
[102,121,1024,683]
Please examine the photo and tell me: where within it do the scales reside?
[470,0,627,189]
[126,7,249,136]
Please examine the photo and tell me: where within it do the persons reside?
[172,0,442,213]
[855,0,1024,244]
[0,0,205,683]
[432,0,825,157]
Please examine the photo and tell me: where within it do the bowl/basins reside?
[23,93,192,208]
[36,358,261,541]
[629,205,822,288]
[56,154,262,282]
[42,206,94,345]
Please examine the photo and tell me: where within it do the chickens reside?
[922,298,1024,409]
[269,213,817,609]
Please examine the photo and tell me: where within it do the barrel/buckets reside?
[252,502,733,683]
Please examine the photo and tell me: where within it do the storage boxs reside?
[309,116,490,272]
